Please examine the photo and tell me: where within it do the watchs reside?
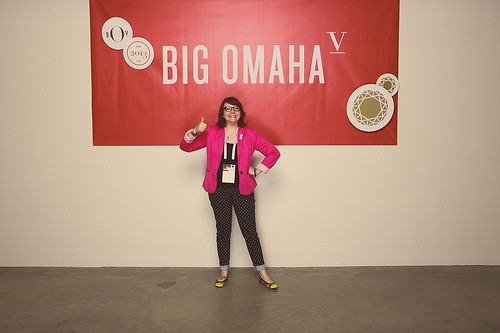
[190,128,198,137]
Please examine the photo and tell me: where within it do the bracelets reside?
[254,167,256,177]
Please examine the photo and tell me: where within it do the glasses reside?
[223,106,240,112]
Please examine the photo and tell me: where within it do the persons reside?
[179,96,281,289]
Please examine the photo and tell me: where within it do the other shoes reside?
[215,276,228,287]
[259,277,278,289]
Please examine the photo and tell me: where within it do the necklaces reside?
[226,126,237,140]
